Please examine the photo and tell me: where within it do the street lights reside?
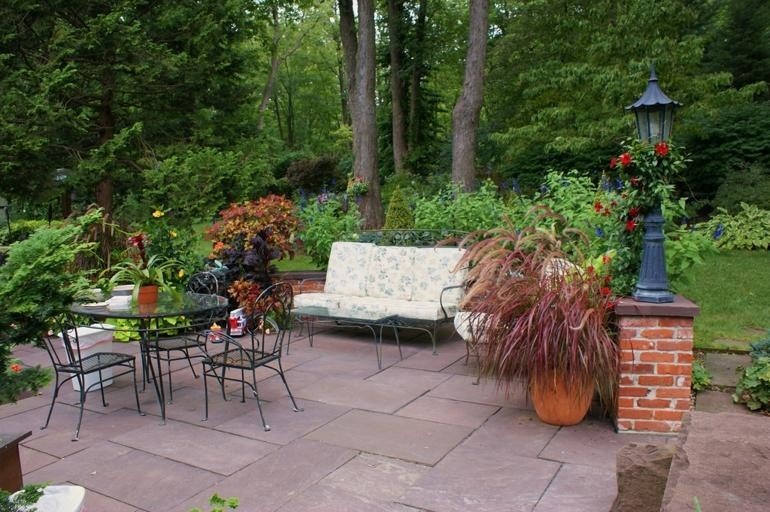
[600,59,701,437]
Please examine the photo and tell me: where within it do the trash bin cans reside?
[57,324,116,392]
